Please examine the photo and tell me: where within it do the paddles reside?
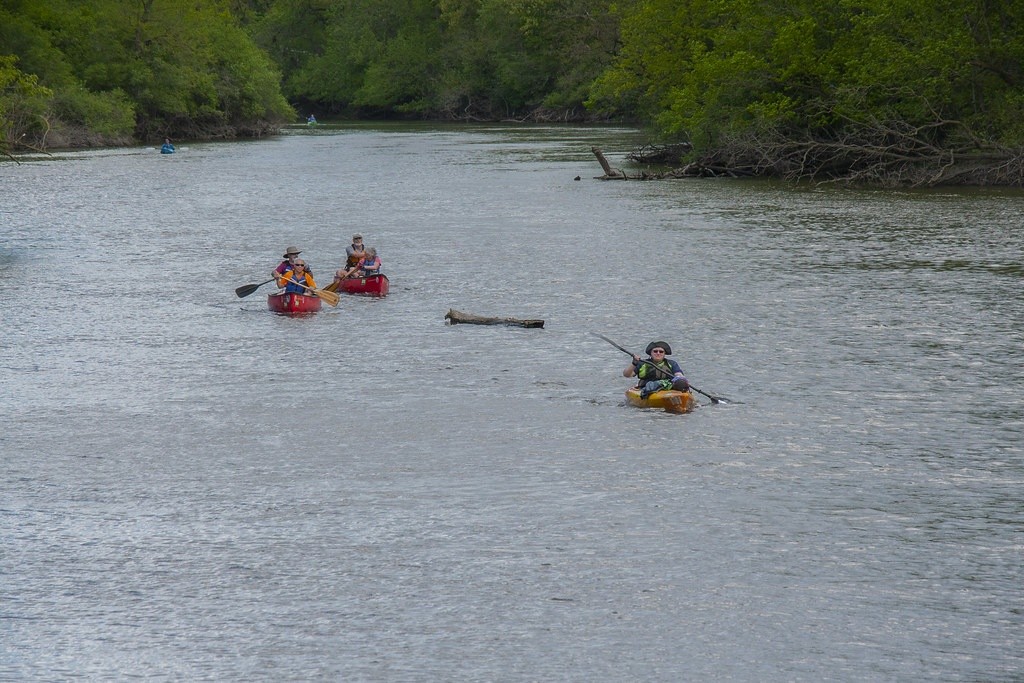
[235,278,276,298]
[147,146,189,151]
[591,326,725,405]
[323,263,360,293]
[272,272,338,308]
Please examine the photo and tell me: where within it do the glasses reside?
[354,237,362,239]
[294,264,305,266]
[290,253,299,255]
[651,349,666,354]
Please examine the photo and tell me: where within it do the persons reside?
[161,139,175,150]
[271,246,313,278]
[353,247,381,278]
[346,235,366,268]
[276,259,317,295]
[623,341,690,391]
[308,114,316,121]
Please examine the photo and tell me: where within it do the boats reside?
[268,292,323,312]
[626,385,693,412]
[333,275,390,295]
[160,146,172,151]
[306,118,316,122]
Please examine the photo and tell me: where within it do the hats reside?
[646,341,672,355]
[283,247,302,258]
[352,233,362,239]
